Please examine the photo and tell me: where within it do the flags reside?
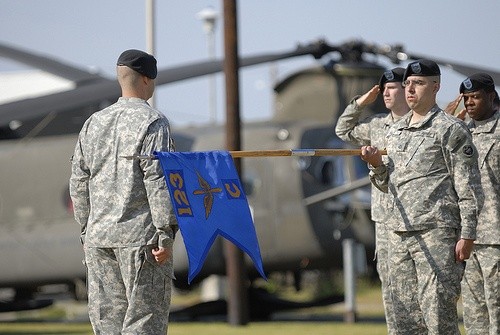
[153,150,267,285]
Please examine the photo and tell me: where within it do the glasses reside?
[401,79,437,87]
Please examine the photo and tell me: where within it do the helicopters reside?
[2,35,500,297]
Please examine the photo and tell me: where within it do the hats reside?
[379,67,406,94]
[403,60,441,81]
[460,73,495,94]
[117,49,157,79]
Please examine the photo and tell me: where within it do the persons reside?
[445,73,500,335]
[360,60,480,335]
[68,49,179,335]
[335,67,414,335]
